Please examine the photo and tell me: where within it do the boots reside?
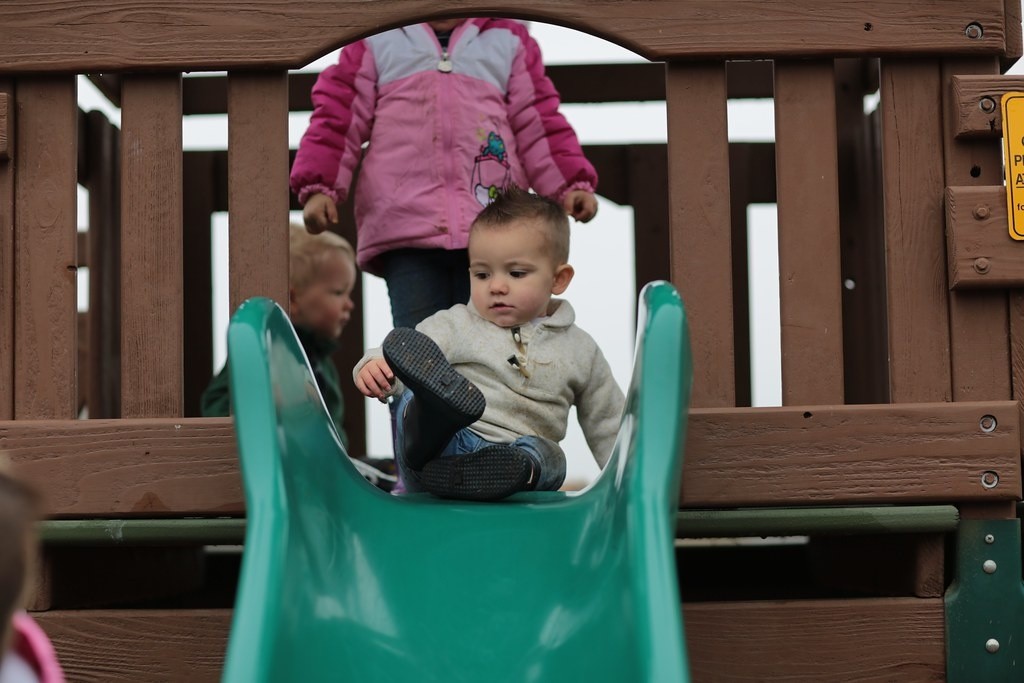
[419,445,541,501]
[381,324,486,472]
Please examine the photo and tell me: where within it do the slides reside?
[221,281,693,683]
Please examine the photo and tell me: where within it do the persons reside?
[192,219,361,476]
[348,188,632,500]
[288,14,603,492]
[2,469,74,683]
